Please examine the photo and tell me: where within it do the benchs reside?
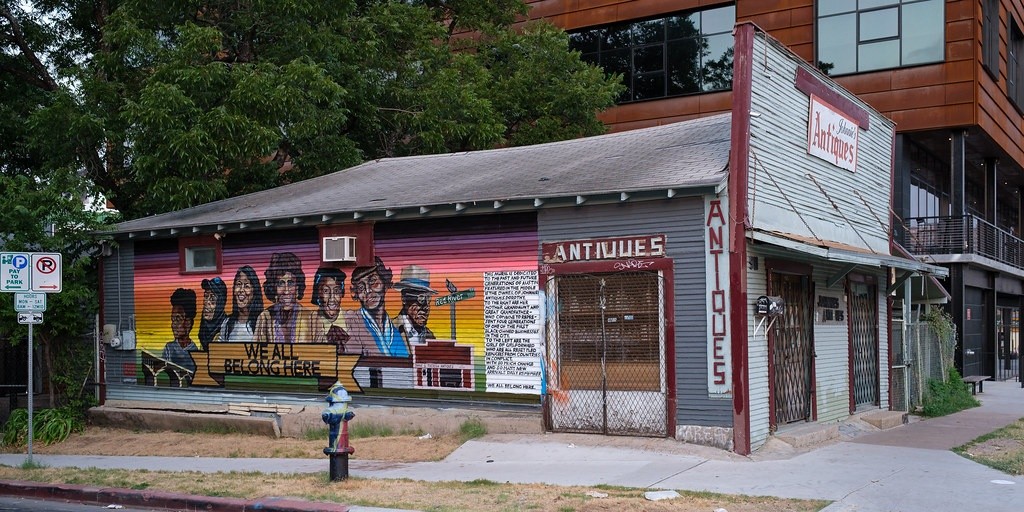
[962,376,991,395]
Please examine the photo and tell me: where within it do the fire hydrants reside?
[322,381,355,482]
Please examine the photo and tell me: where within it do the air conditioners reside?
[323,236,356,262]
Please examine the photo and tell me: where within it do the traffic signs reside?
[0,252,30,292]
[30,252,62,293]
[14,293,46,324]
